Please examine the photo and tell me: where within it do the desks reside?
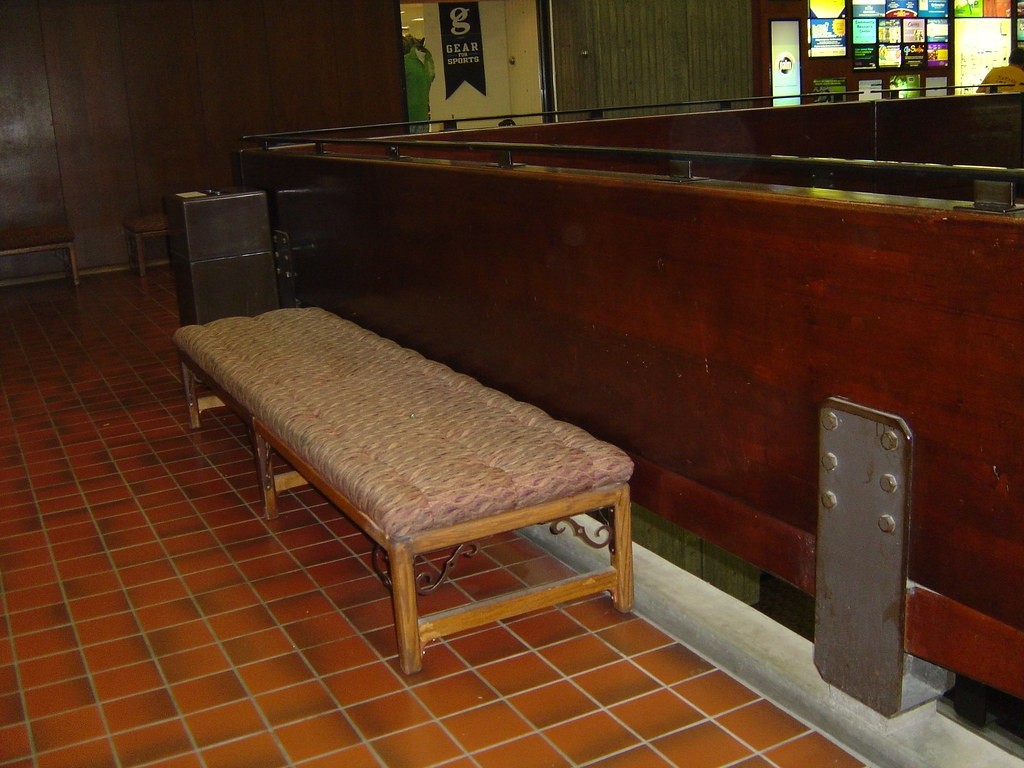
[163,185,272,383]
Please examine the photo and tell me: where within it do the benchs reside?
[171,305,636,675]
[122,212,169,278]
[0,223,81,287]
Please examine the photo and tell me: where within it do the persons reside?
[976,48,1024,93]
[404,35,435,133]
